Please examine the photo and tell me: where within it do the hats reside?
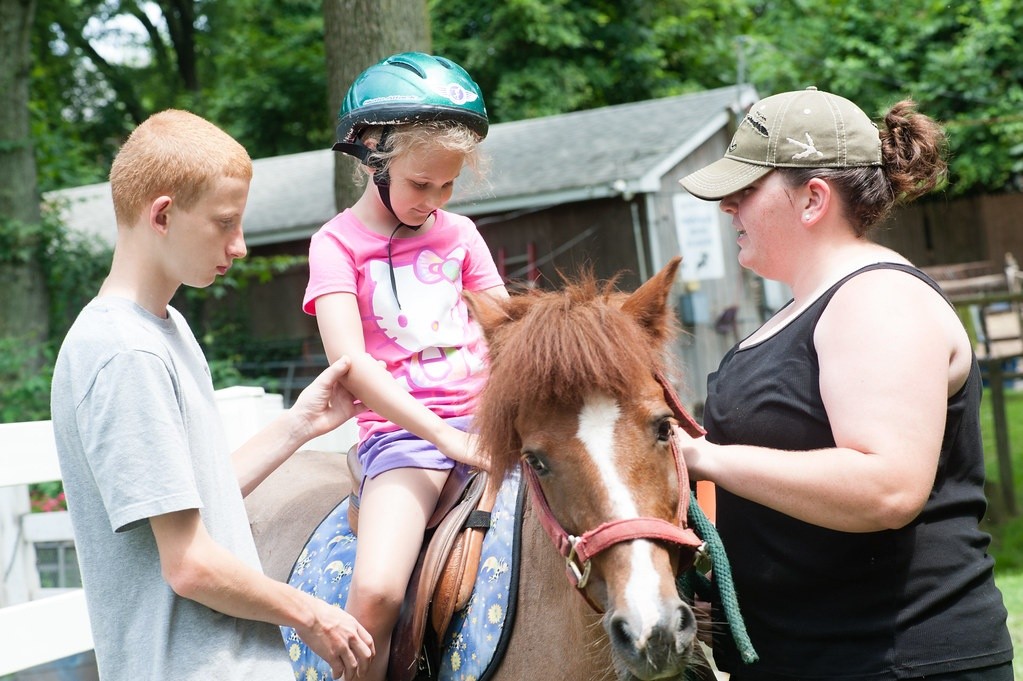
[679,85,883,201]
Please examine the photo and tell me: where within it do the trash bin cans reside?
[969,301,1017,390]
[681,290,709,328]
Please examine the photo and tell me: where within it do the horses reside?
[239,255,730,681]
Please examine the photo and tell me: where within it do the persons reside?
[51,108,389,681]
[673,87,1015,681]
[302,51,514,681]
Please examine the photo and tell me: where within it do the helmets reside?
[335,51,490,139]
